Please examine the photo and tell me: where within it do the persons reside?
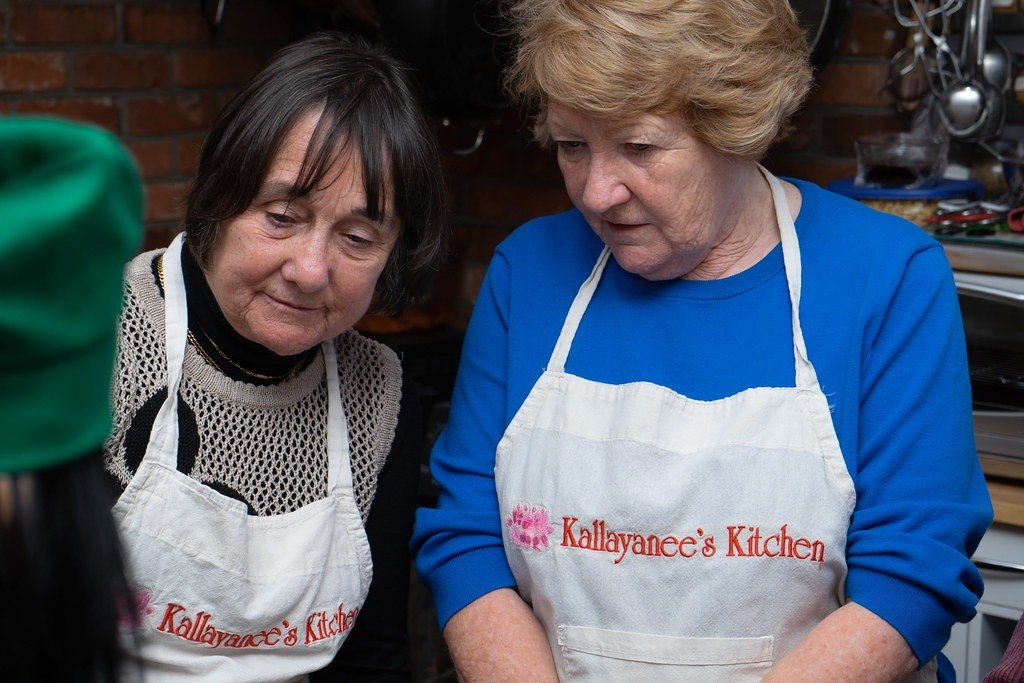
[408,1,993,683]
[95,34,438,683]
[2,118,155,682]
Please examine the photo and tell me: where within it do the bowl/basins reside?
[830,178,977,231]
[855,133,948,188]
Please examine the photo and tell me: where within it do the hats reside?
[0,118,145,472]
